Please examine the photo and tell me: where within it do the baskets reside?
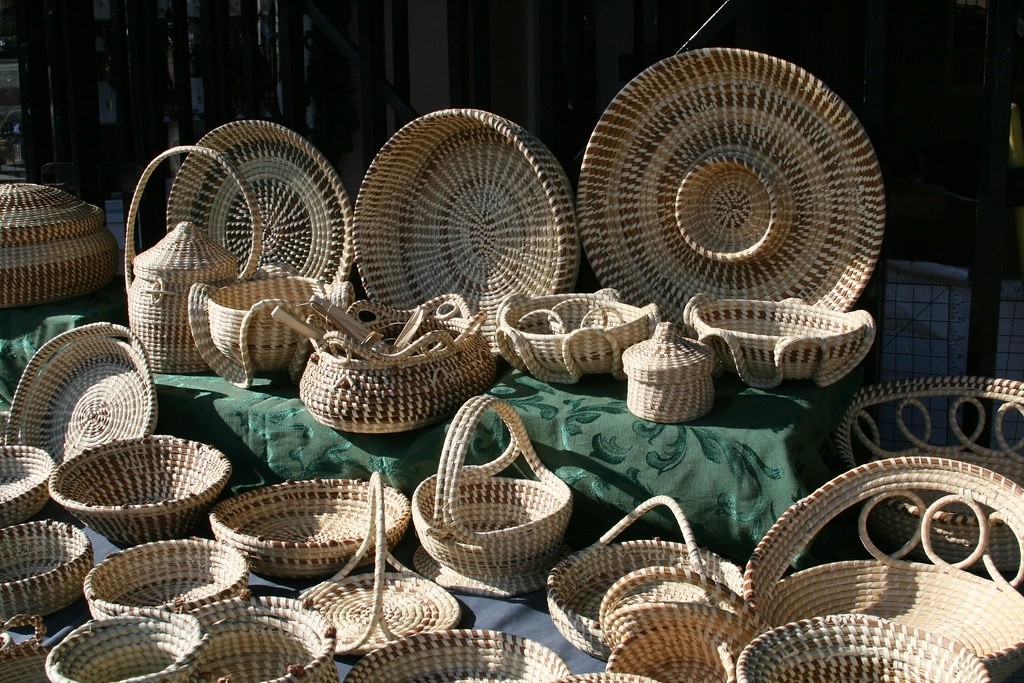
[124,146,262,374]
[5,322,158,466]
[188,262,356,389]
[684,292,876,388]
[577,48,885,360]
[299,293,496,433]
[0,395,572,683]
[353,108,580,356]
[166,120,354,282]
[546,377,1024,683]
[495,287,662,385]
